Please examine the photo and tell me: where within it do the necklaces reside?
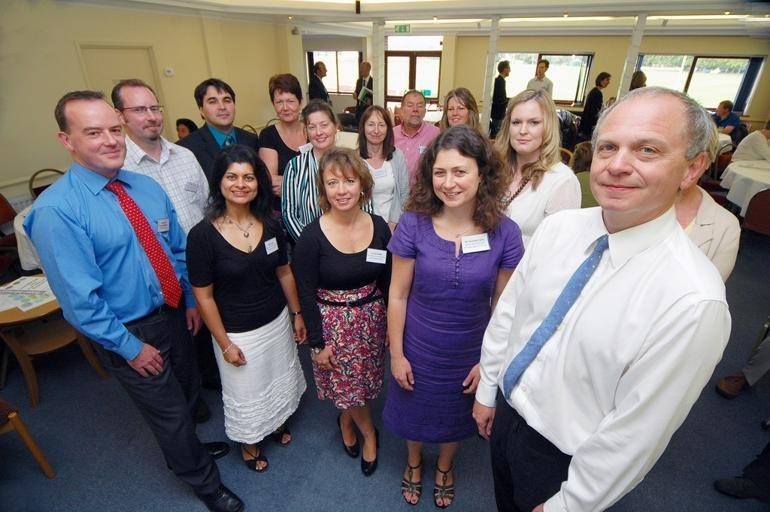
[452,223,475,237]
[224,212,254,237]
[498,173,532,210]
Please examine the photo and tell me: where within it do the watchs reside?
[310,347,326,354]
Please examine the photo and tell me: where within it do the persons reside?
[711,101,742,144]
[382,123,526,508]
[186,144,306,471]
[259,73,310,214]
[716,333,770,399]
[353,62,373,106]
[578,72,613,141]
[713,440,770,512]
[23,90,246,512]
[473,87,732,512]
[306,62,333,108]
[357,105,409,234]
[527,60,553,97]
[109,78,221,423]
[281,99,374,239]
[174,79,260,201]
[676,118,742,284]
[175,119,198,141]
[296,147,392,477]
[435,88,480,132]
[628,71,647,92]
[494,89,583,252]
[391,90,441,188]
[490,61,511,138]
[730,119,770,161]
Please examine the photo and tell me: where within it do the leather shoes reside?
[168,441,231,471]
[194,483,244,511]
[194,397,211,422]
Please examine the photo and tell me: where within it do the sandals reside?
[400,453,424,507]
[271,426,293,446]
[433,455,456,510]
[240,443,270,473]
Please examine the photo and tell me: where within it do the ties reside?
[361,78,367,107]
[222,136,235,145]
[106,180,181,309]
[502,234,609,401]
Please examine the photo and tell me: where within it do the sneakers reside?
[712,475,767,500]
[715,371,752,400]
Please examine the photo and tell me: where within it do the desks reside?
[721,158,770,235]
[0,275,110,407]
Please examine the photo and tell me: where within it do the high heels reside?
[336,412,360,459]
[360,425,382,477]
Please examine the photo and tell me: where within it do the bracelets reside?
[292,311,301,316]
[222,343,233,355]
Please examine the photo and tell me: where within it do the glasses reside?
[122,104,162,115]
